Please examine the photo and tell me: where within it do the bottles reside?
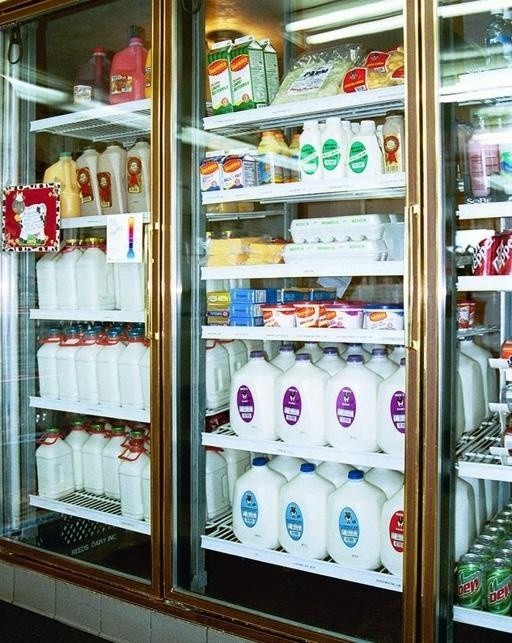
[456,3,512,198]
[257,108,406,184]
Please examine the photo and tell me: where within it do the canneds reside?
[471,228,512,276]
[456,504,512,617]
